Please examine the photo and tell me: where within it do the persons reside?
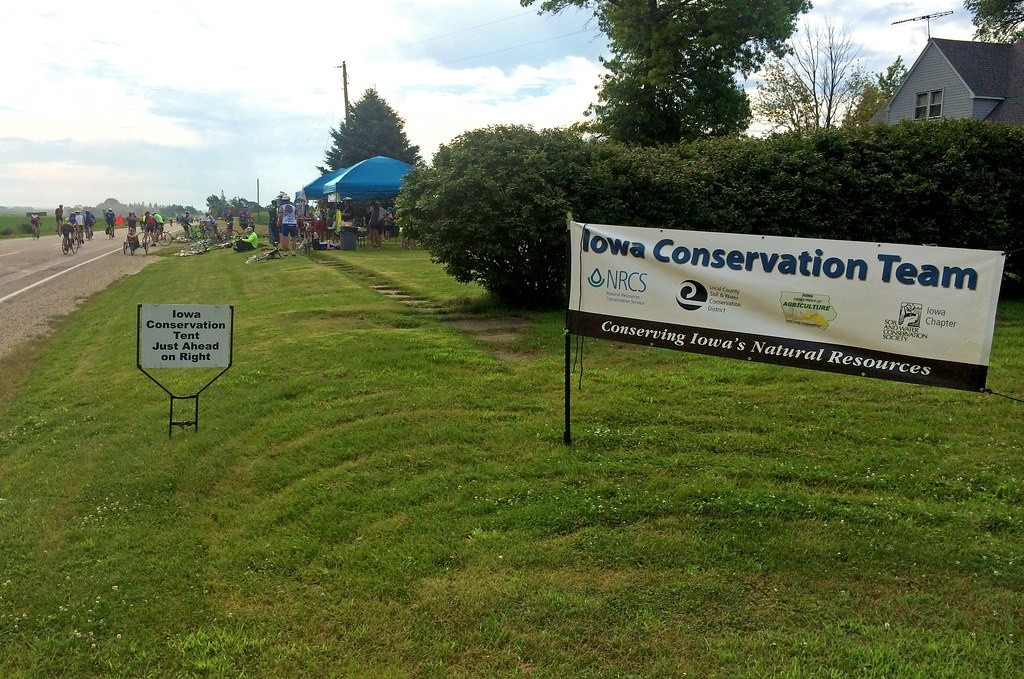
[226,205,236,241]
[205,211,216,224]
[238,207,248,238]
[55,205,63,232]
[31,211,41,238]
[127,211,172,246]
[235,227,258,252]
[61,211,96,250]
[364,202,388,240]
[105,208,115,237]
[268,194,298,256]
[343,197,353,213]
[176,211,196,238]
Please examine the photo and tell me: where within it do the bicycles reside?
[85,222,172,254]
[30,222,42,240]
[62,227,79,255]
[175,225,242,242]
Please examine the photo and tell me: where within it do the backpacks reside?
[107,213,114,221]
[31,215,39,222]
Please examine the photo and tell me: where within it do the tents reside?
[295,167,346,218]
[323,155,414,208]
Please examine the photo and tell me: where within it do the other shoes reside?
[297,244,305,250]
[284,252,288,256]
[291,251,297,255]
[150,244,156,246]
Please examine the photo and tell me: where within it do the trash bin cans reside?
[339,226,359,251]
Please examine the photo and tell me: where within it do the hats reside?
[128,212,132,215]
[185,211,189,214]
[75,210,80,214]
[387,207,394,212]
[109,208,113,210]
[281,195,290,201]
[246,227,253,232]
